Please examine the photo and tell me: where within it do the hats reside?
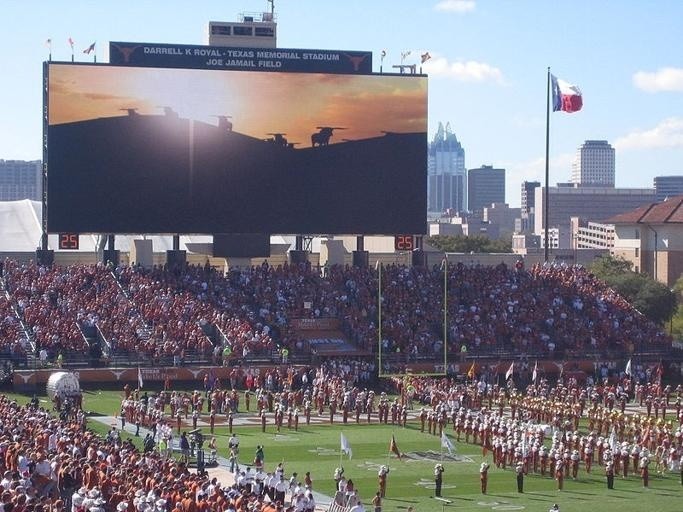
[481,462,487,467]
[117,502,128,511]
[435,464,441,468]
[380,465,386,470]
[421,400,683,466]
[78,487,107,505]
[176,502,182,506]
[335,468,341,473]
[134,489,167,506]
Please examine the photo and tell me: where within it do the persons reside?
[1,255,683,512]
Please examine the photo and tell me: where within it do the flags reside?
[379,49,432,64]
[45,37,99,56]
[551,73,584,113]
